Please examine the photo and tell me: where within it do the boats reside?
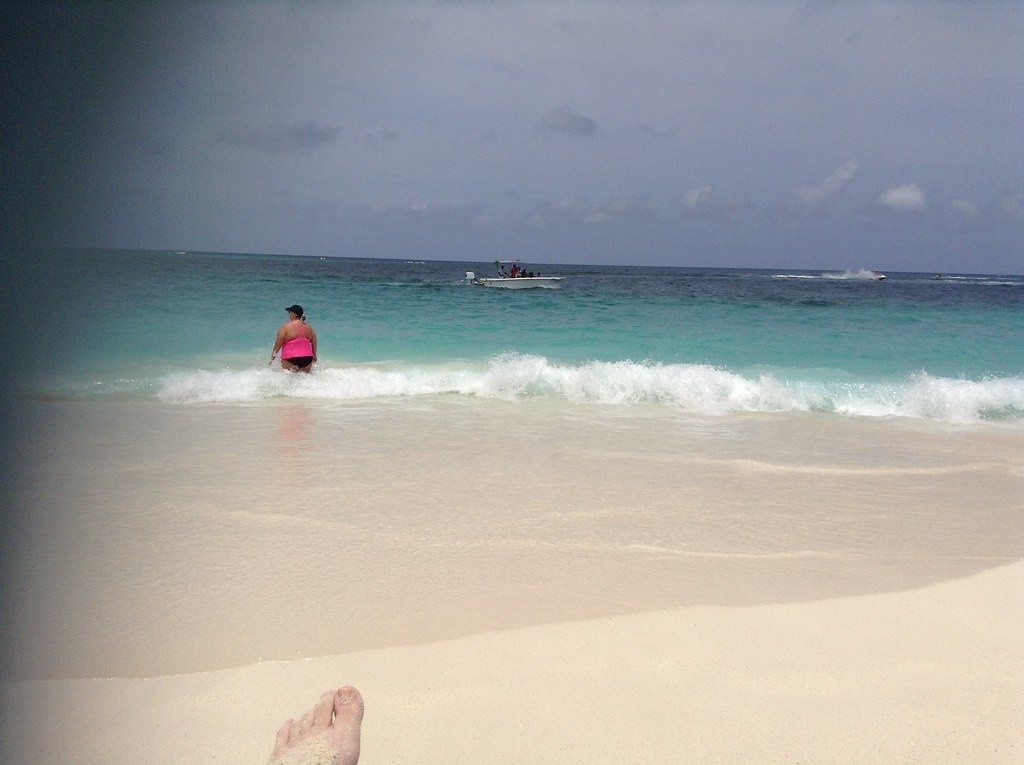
[466,271,567,290]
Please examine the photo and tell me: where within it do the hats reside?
[285,305,304,316]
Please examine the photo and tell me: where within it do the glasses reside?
[289,311,293,314]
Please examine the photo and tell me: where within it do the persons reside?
[497,264,541,278]
[269,304,317,373]
[269,684,365,765]
